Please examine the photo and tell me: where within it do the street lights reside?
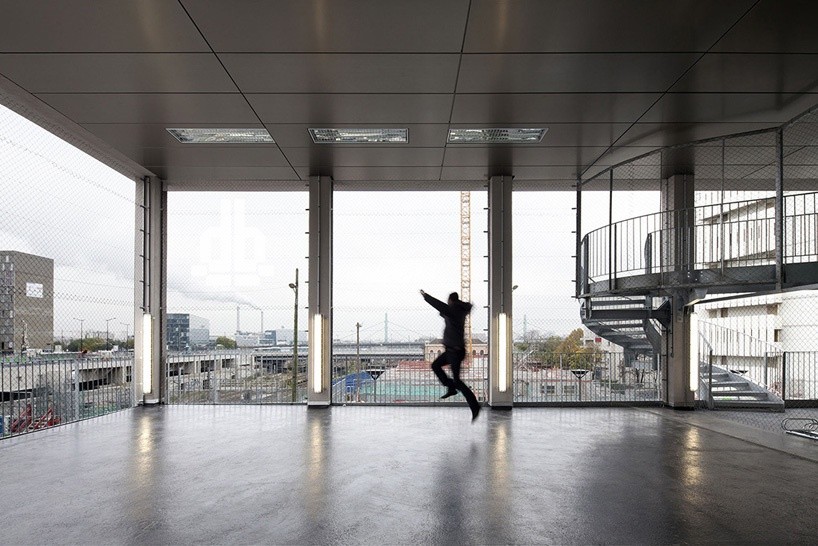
[364,368,386,402]
[356,322,362,400]
[119,322,130,352]
[106,317,117,351]
[571,370,590,403]
[72,317,85,352]
[289,268,300,400]
[730,368,749,400]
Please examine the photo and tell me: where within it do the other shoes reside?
[472,404,481,420]
[441,390,458,399]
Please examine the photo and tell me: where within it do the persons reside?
[421,289,481,423]
